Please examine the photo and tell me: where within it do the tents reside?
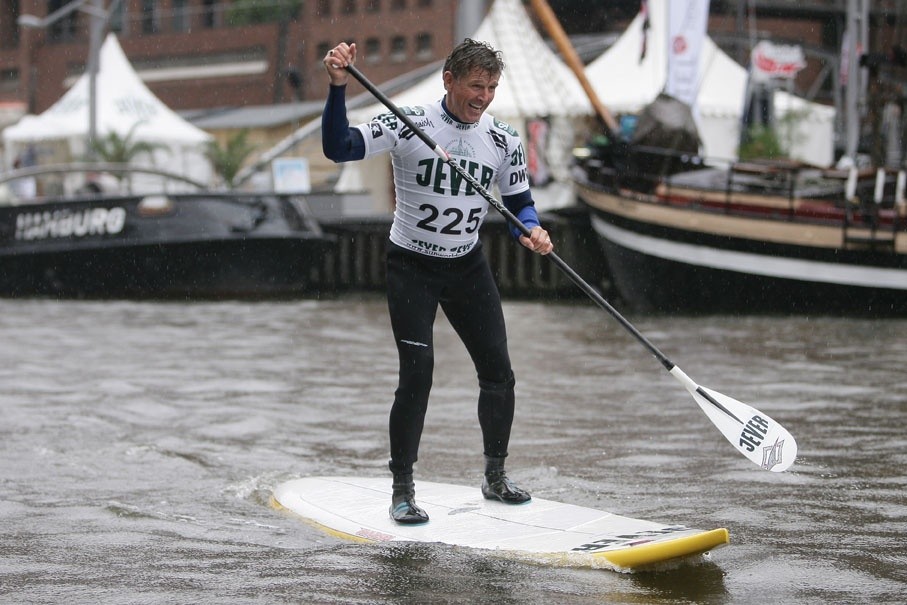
[0,33,216,201]
[338,0,587,189]
[574,12,835,170]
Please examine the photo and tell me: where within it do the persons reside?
[11,159,37,198]
[77,172,101,193]
[323,38,553,523]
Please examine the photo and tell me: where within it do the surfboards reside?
[266,474,731,569]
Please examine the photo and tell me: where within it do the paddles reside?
[330,54,799,472]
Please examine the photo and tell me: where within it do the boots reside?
[482,452,531,502]
[391,472,429,523]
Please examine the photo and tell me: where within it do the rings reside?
[329,50,334,56]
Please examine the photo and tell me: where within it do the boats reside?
[0,0,907,322]
